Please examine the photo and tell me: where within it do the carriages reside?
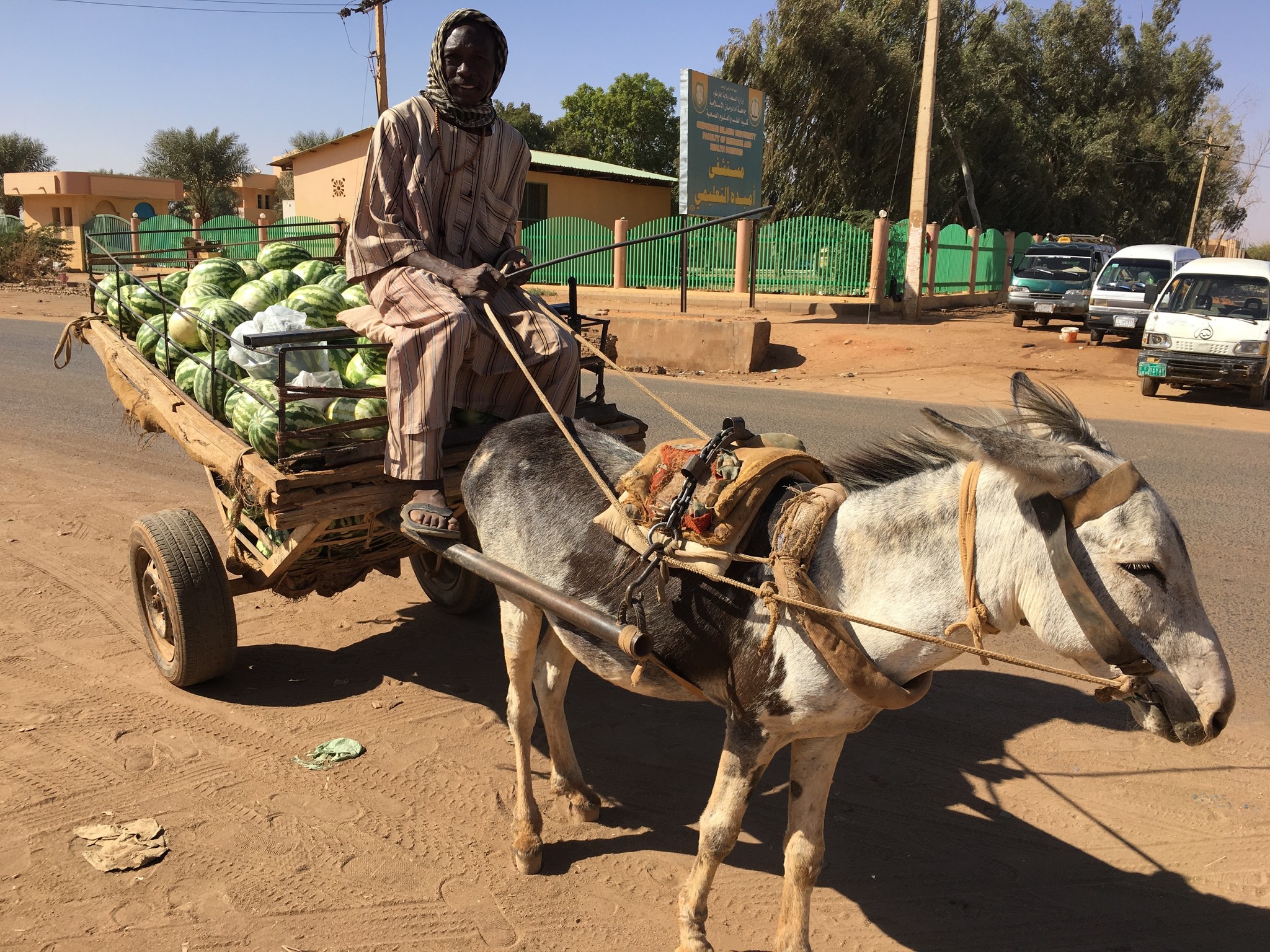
[78,221,1236,952]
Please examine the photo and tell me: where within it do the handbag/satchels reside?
[290,369,342,413]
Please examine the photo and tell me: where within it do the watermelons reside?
[95,242,492,559]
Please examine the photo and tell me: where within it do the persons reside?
[347,10,580,541]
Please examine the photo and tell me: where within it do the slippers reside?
[399,502,461,540]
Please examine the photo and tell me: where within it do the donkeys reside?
[464,370,1236,952]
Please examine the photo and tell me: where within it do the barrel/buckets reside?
[1061,327,1079,342]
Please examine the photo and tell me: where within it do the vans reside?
[1006,233,1117,329]
[1088,244,1202,344]
[1136,258,1270,408]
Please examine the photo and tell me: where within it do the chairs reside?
[1191,294,1263,318]
[1077,258,1090,271]
[1047,258,1062,269]
